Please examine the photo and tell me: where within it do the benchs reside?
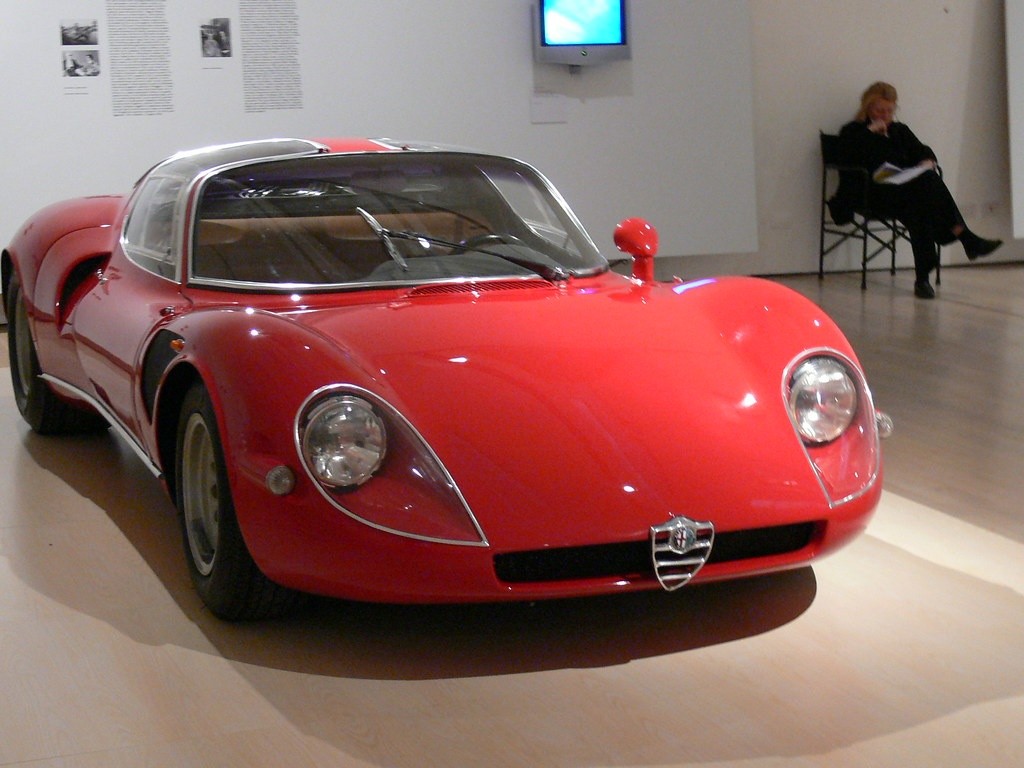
[195,208,495,285]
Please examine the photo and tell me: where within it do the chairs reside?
[817,129,941,289]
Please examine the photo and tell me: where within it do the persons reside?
[829,81,1002,298]
[73,24,88,40]
[204,31,229,55]
[63,54,99,76]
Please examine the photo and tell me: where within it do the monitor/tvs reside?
[532,0,633,66]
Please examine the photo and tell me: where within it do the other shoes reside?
[914,281,935,298]
[958,226,1003,262]
